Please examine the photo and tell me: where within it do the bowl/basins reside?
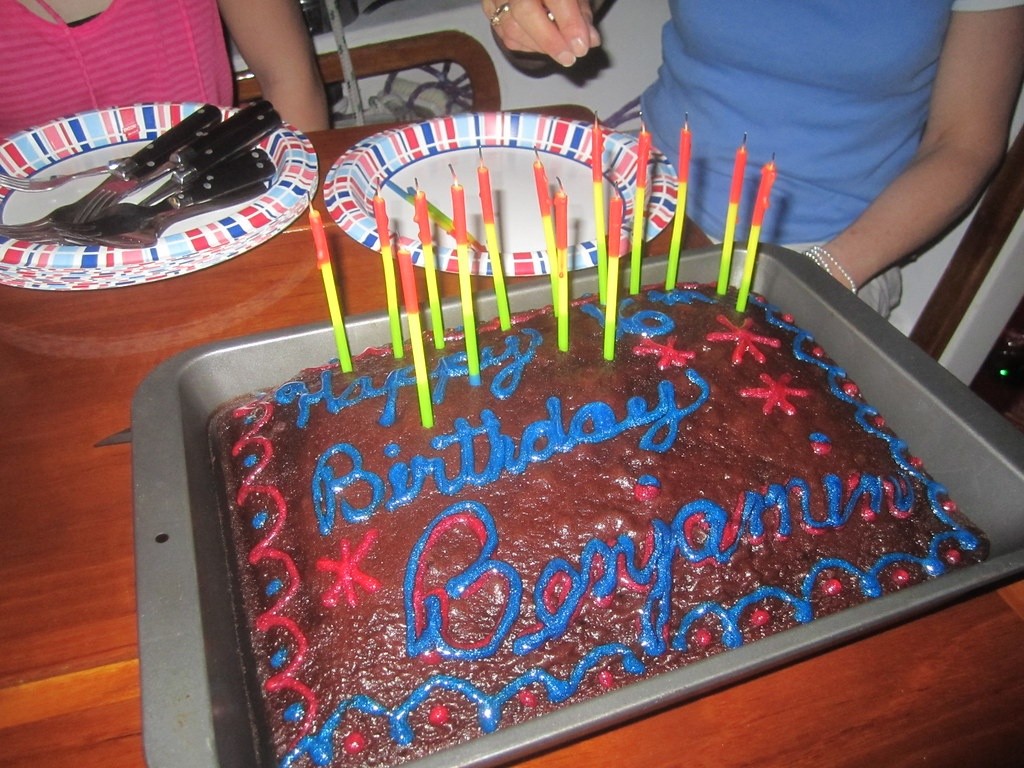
[299,0,357,38]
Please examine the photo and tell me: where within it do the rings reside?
[489,3,510,26]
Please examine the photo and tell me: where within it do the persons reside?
[0,0,332,144]
[473,0,1022,304]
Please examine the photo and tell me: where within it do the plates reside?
[322,112,677,277]
[0,100,320,289]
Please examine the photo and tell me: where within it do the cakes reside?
[217,279,990,766]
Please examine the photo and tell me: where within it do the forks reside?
[1,100,282,250]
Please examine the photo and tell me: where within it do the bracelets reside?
[803,246,858,297]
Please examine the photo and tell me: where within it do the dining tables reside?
[0,104,1024,768]
